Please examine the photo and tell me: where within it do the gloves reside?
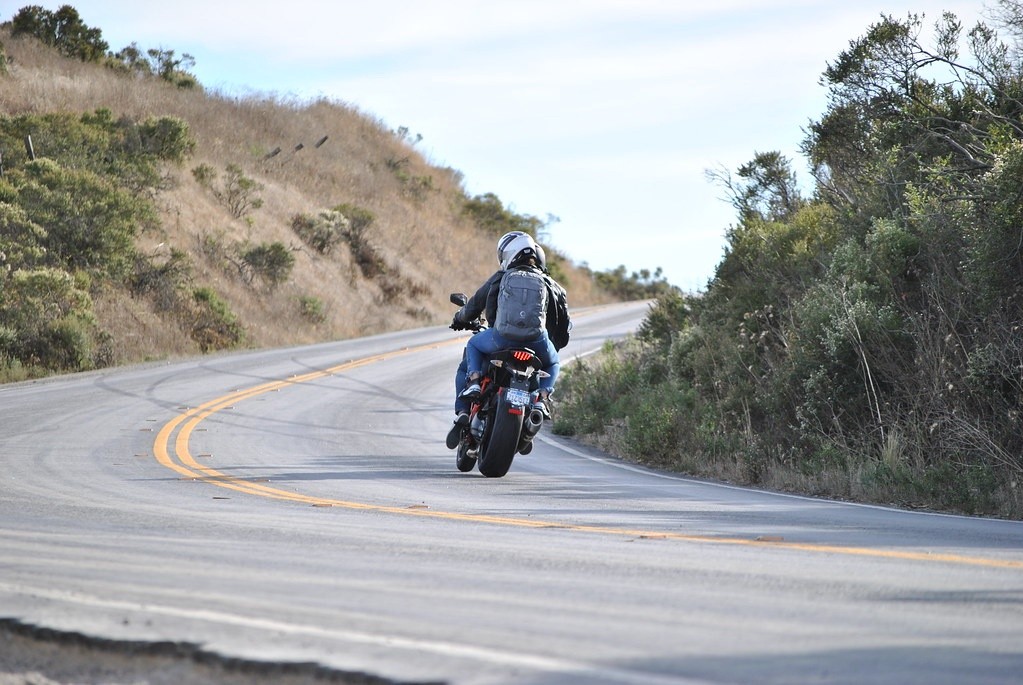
[453,312,464,331]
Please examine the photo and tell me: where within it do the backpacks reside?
[494,268,549,342]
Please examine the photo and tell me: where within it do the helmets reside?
[534,242,546,272]
[496,230,537,271]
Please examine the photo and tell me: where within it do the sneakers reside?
[457,377,481,401]
[533,398,551,420]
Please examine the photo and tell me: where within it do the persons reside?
[446,231,570,456]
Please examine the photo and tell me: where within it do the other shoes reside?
[446,412,469,449]
[519,440,533,455]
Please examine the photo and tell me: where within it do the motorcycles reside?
[447,291,557,480]
